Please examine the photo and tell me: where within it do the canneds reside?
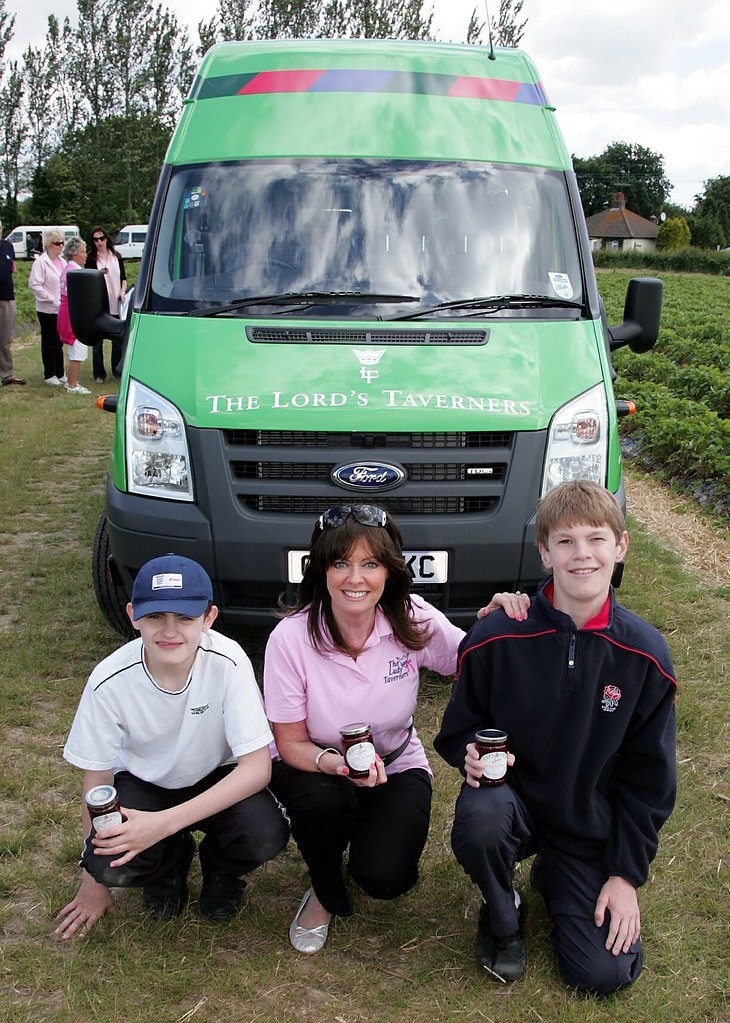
[85,784,123,833]
[339,722,379,779]
[475,728,507,787]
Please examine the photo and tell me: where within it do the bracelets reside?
[316,748,341,775]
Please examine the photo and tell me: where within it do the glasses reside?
[51,241,65,246]
[311,504,396,547]
[93,236,105,242]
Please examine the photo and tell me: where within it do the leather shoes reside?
[199,841,243,918]
[142,833,196,920]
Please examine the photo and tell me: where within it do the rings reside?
[516,591,521,595]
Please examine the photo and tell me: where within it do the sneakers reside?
[475,888,526,981]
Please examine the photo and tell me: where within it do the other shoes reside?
[96,376,104,383]
[44,376,67,385]
[2,376,27,385]
[289,886,331,954]
[64,381,92,395]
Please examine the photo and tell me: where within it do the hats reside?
[131,553,213,622]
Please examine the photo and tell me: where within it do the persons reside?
[0,222,26,386]
[55,554,291,940]
[29,232,69,385]
[58,238,93,394]
[435,482,677,1000]
[26,234,36,259]
[263,504,529,954]
[85,226,128,383]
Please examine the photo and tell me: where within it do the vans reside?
[5,226,80,259]
[113,225,149,262]
[66,37,663,641]
